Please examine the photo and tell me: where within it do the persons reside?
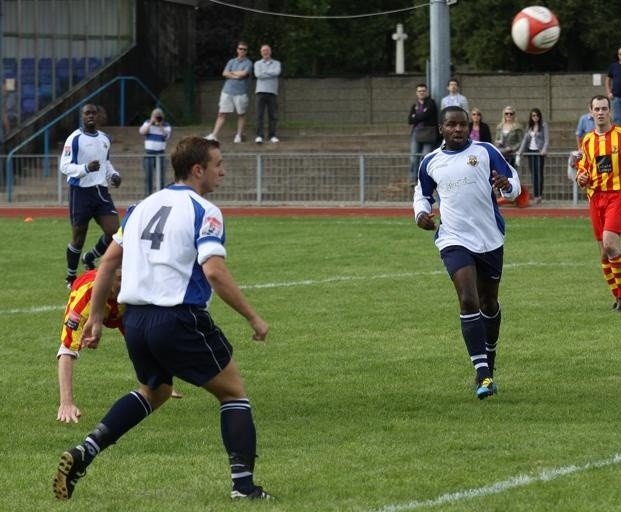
[55,265,183,423]
[203,40,252,143]
[467,108,493,144]
[52,137,275,499]
[518,108,551,202]
[138,107,172,199]
[0,76,17,132]
[605,45,621,126]
[495,107,525,167]
[413,106,522,399]
[59,102,120,289]
[576,104,599,150]
[575,95,621,311]
[408,83,440,187]
[440,80,469,115]
[253,45,281,143]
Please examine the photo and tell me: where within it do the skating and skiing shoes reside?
[51,445,86,502]
[230,485,275,505]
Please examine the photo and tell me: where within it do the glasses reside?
[417,90,426,93]
[503,112,513,115]
[472,113,481,116]
[531,114,539,117]
[238,47,247,51]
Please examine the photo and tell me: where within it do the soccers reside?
[510,6,561,54]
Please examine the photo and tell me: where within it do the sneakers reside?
[203,134,217,139]
[477,375,499,399]
[234,136,242,142]
[270,138,278,142]
[81,253,96,272]
[256,136,262,143]
[64,278,75,290]
